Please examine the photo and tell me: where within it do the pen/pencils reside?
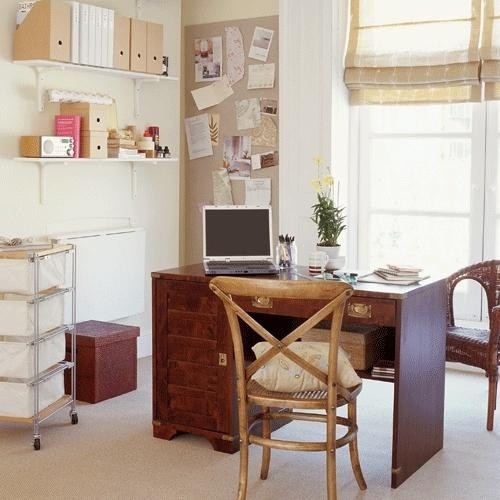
[278,235,294,267]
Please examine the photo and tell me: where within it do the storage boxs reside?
[300,320,387,373]
[14,1,70,62]
[63,317,141,406]
[113,14,166,74]
[57,101,118,160]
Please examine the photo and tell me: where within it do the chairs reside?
[207,273,371,498]
[445,261,500,432]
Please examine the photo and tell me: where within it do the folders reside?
[70,2,114,69]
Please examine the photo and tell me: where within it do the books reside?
[373,352,395,370]
[0,239,54,252]
[386,261,423,272]
[374,271,423,282]
[373,369,395,375]
[379,267,420,276]
[376,270,422,278]
[357,270,433,287]
[371,369,394,380]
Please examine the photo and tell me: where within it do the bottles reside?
[157,146,171,158]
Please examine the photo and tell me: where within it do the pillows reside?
[248,341,363,392]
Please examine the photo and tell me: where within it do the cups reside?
[309,251,329,278]
[275,241,298,269]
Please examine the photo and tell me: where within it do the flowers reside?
[310,154,347,246]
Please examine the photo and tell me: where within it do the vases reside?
[315,242,341,258]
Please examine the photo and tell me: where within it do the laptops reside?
[202,204,281,275]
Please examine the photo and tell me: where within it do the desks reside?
[149,261,449,489]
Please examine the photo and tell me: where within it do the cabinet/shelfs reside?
[10,59,180,163]
[1,244,78,451]
